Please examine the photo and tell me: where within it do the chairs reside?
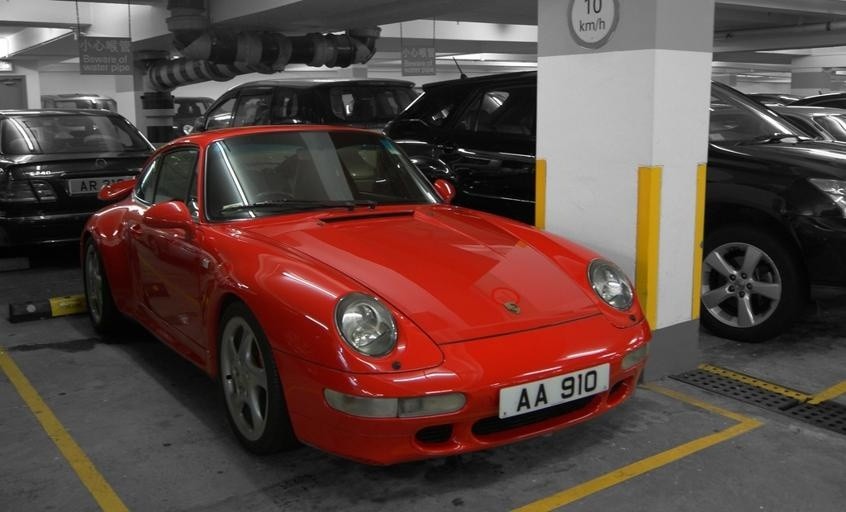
[251,130,376,214]
[458,106,536,155]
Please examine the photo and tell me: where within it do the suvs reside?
[373,68,846,341]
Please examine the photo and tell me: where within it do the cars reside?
[82,123,653,466]
[711,87,846,143]
[174,96,216,133]
[1,106,157,267]
[41,93,117,111]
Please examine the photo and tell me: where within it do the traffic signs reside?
[567,0,618,49]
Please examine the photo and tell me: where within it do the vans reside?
[174,78,417,194]
